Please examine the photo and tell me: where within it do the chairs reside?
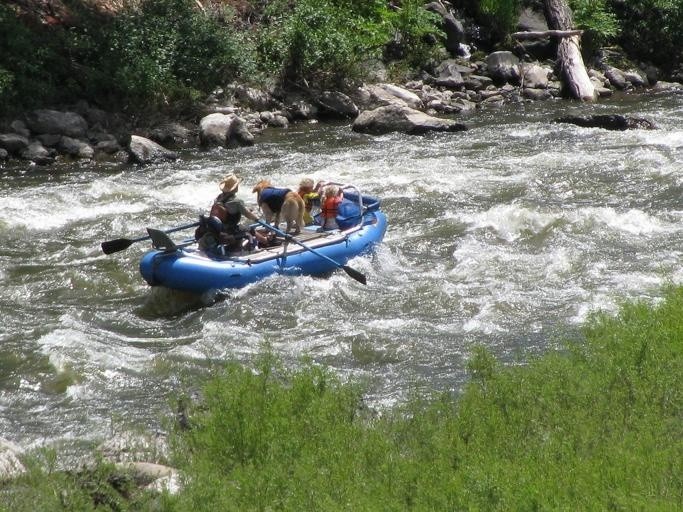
[207,203,255,255]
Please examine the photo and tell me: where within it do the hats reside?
[218,173,243,193]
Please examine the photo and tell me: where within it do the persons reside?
[296,178,325,225]
[209,174,283,247]
[319,186,344,230]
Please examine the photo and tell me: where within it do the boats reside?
[138,179,388,289]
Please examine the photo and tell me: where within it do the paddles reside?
[257,221,366,286]
[101,221,200,255]
